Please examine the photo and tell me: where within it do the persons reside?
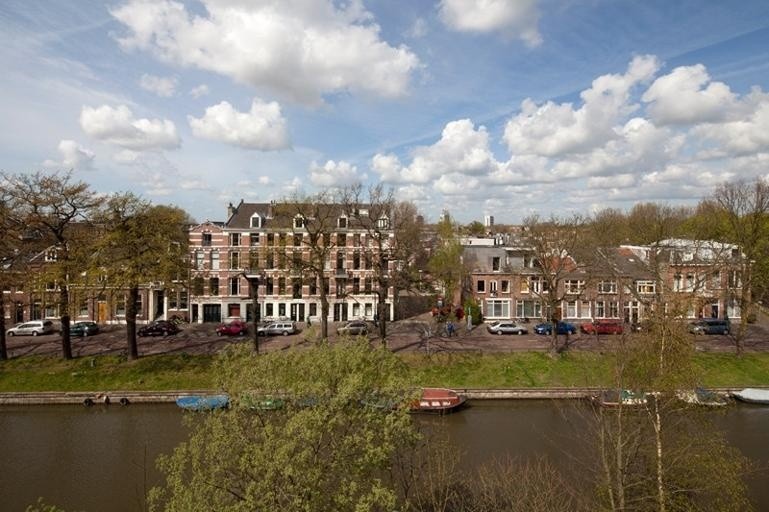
[445,321,448,335]
[448,321,454,338]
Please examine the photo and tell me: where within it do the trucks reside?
[433,297,447,316]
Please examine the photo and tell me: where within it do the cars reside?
[534,320,576,336]
[216,320,247,337]
[487,320,529,336]
[137,320,177,337]
[337,320,367,335]
[59,321,98,336]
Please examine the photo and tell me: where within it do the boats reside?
[679,389,730,407]
[592,389,648,408]
[241,392,283,410]
[369,387,466,412]
[733,388,769,403]
[174,393,229,410]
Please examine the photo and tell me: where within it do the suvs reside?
[582,319,624,334]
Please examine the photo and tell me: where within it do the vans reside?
[6,319,52,336]
[689,318,730,336]
[257,320,297,336]
